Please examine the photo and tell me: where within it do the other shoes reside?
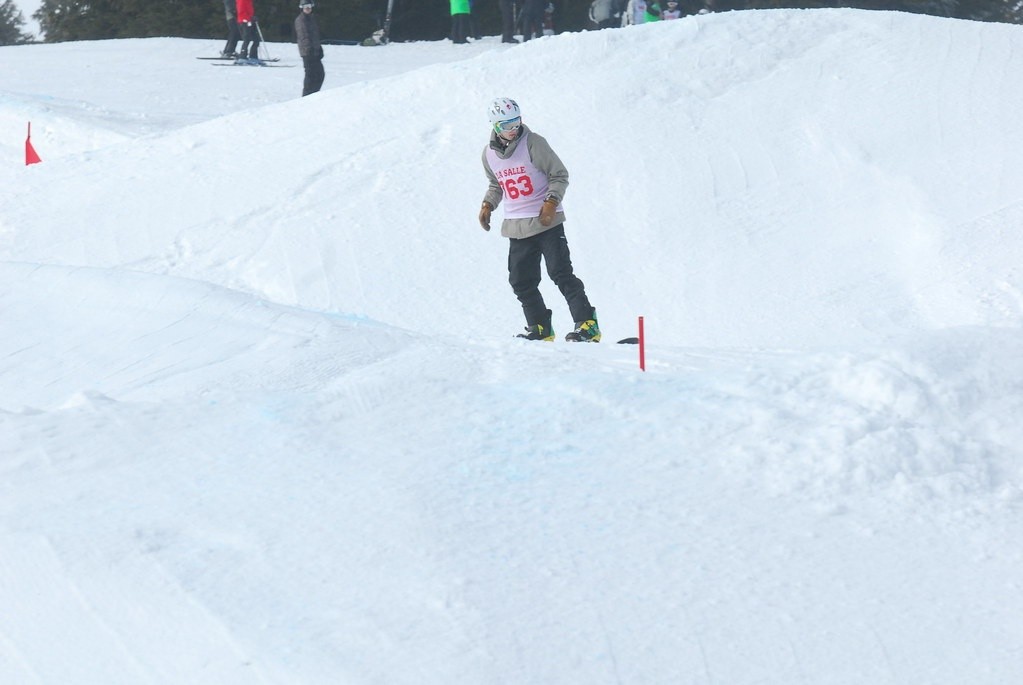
[250,58,262,63]
[238,58,253,64]
[232,52,239,57]
[221,53,231,58]
[502,38,519,43]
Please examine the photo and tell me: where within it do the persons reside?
[219,0,263,64]
[479,96,603,342]
[449,0,723,44]
[294,0,325,97]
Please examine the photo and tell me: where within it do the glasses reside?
[496,116,521,132]
[302,3,312,9]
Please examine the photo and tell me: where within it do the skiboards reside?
[195,56,299,68]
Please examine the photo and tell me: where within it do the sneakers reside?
[517,309,555,340]
[565,307,601,342]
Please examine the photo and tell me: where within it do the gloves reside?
[319,46,323,59]
[479,201,493,231]
[539,200,558,226]
[303,56,311,72]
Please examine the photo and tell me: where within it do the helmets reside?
[299,0,314,8]
[487,97,520,134]
